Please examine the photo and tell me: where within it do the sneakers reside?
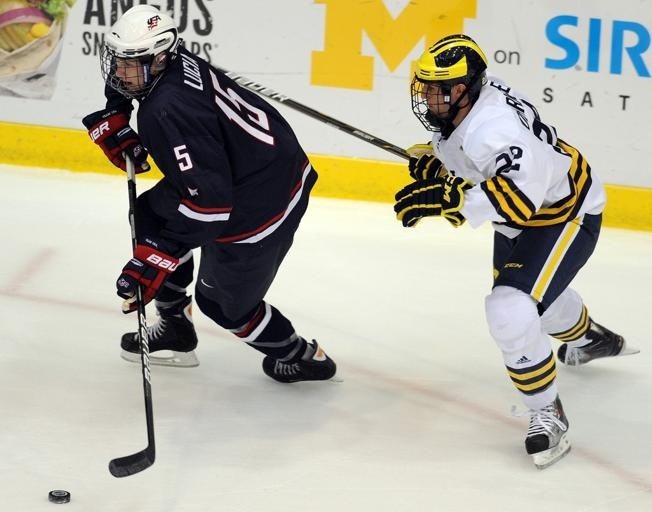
[558,318,624,364]
[525,397,569,454]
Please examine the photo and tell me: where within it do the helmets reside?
[100,5,178,98]
[411,34,487,132]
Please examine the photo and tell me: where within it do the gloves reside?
[394,177,472,228]
[82,109,150,174]
[116,237,179,313]
[409,141,450,178]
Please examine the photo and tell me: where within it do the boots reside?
[263,339,336,382]
[121,297,198,353]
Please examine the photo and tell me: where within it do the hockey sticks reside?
[177,46,415,162]
[110,155,156,477]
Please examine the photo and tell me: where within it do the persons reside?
[394,33,624,453]
[80,6,338,385]
